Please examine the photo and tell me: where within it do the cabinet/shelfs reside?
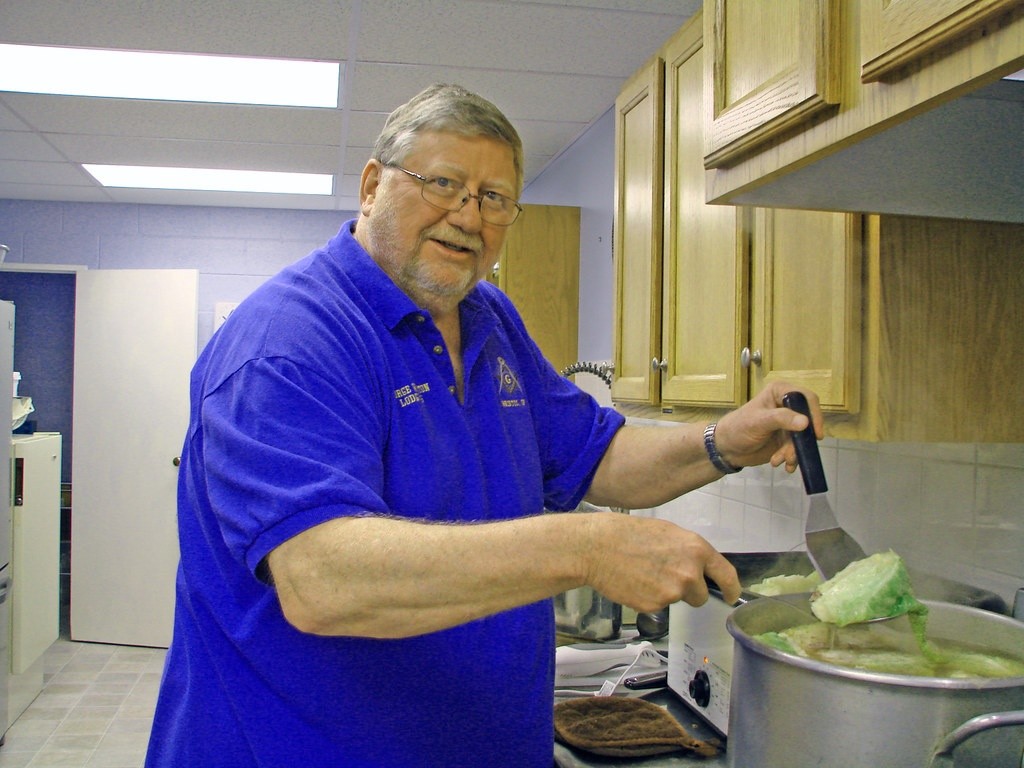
[482,204,581,384]
[611,0,1024,441]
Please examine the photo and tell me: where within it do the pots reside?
[724,590,1024,768]
[665,543,1008,749]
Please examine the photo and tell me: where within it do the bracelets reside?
[704,423,745,474]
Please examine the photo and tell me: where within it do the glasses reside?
[390,163,522,225]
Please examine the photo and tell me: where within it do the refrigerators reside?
[0,299,15,745]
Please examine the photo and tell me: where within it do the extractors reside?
[704,55,1024,223]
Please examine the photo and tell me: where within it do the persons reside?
[143,78,826,768]
